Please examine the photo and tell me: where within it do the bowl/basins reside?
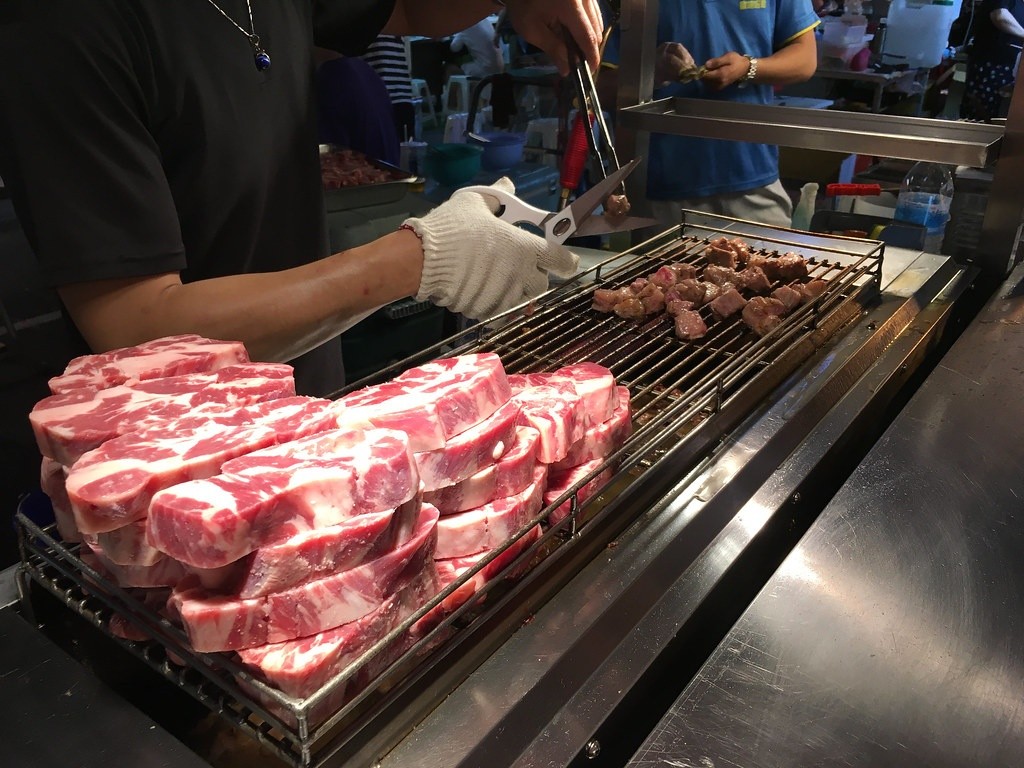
[465,131,528,171]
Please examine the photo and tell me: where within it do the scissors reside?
[449,156,660,245]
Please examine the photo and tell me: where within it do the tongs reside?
[567,36,625,211]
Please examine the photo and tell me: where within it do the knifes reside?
[826,183,899,195]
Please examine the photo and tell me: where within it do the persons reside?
[1,0,604,365]
[449,16,505,76]
[362,34,418,175]
[596,0,822,229]
[959,0,1024,123]
[311,44,401,172]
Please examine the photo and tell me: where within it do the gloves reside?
[654,42,697,90]
[398,175,580,330]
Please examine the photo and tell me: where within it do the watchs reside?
[740,54,758,81]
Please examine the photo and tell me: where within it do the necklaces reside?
[209,0,271,72]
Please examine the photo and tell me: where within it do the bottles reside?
[894,161,954,256]
